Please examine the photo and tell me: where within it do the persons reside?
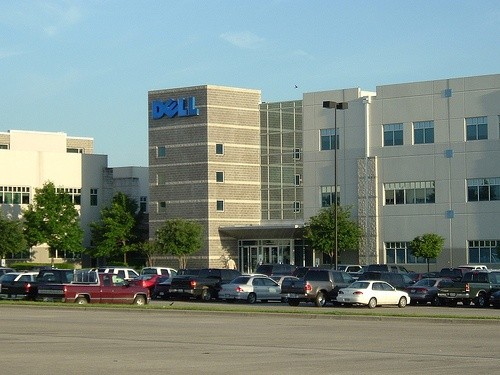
[227,257,236,269]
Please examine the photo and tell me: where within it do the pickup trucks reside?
[37,271,152,307]
[281,268,355,308]
[0,268,79,302]
[170,268,244,302]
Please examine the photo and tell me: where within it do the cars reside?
[270,276,300,291]
[357,270,416,291]
[0,271,40,284]
[337,280,411,309]
[333,264,366,280]
[438,270,500,307]
[406,277,455,305]
[292,266,324,280]
[130,275,168,291]
[476,286,500,307]
[0,267,16,277]
[217,274,284,304]
[88,267,140,283]
[410,271,439,285]
[255,264,296,279]
[152,274,174,301]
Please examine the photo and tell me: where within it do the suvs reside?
[458,265,488,272]
[438,267,469,282]
[365,264,409,274]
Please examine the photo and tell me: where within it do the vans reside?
[141,267,179,285]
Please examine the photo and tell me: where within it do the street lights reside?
[323,101,349,271]
